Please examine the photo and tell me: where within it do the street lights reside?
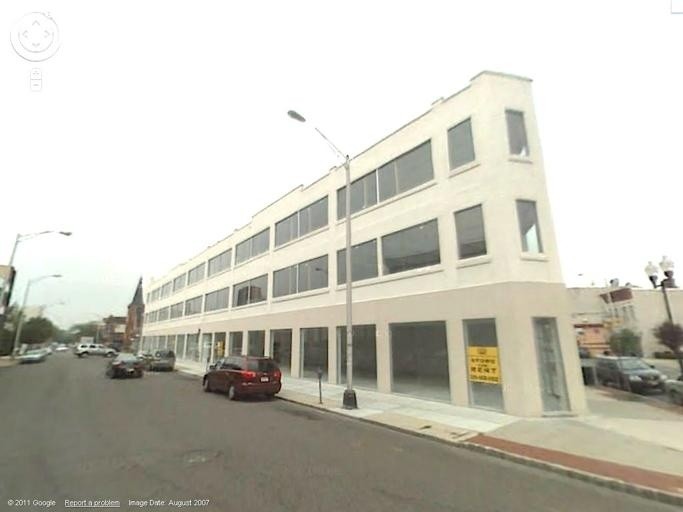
[286,106,358,413]
[6,229,77,359]
[644,254,683,381]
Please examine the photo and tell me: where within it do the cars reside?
[13,340,177,381]
[203,353,282,402]
[577,346,683,408]
